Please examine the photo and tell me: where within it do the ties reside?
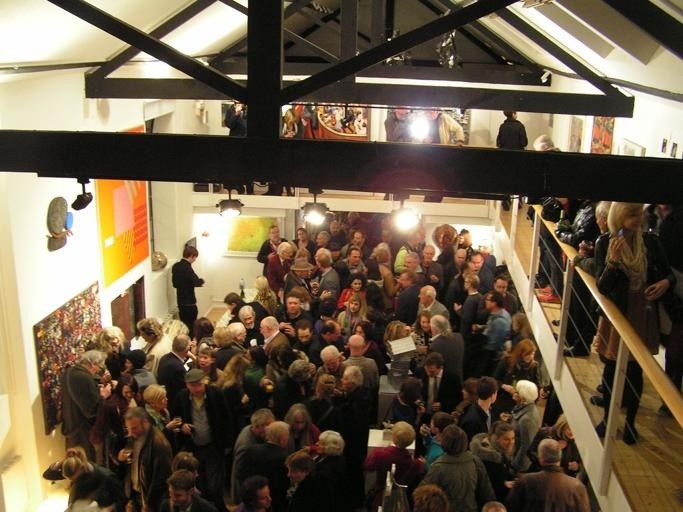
[433,377,438,403]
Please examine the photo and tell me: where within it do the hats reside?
[184,369,204,383]
[290,259,314,271]
[432,225,458,249]
[126,349,146,370]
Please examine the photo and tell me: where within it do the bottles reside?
[239,279,244,289]
[240,290,244,298]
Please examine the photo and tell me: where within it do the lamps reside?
[213,182,412,233]
[69,177,91,210]
[311,106,352,128]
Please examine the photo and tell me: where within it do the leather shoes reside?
[530,274,636,446]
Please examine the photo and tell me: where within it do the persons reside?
[222,99,256,195]
[529,133,683,444]
[44,213,599,512]
[383,105,420,203]
[281,108,305,195]
[494,109,527,208]
[418,108,466,203]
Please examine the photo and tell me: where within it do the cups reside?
[173,416,181,432]
[123,446,133,465]
